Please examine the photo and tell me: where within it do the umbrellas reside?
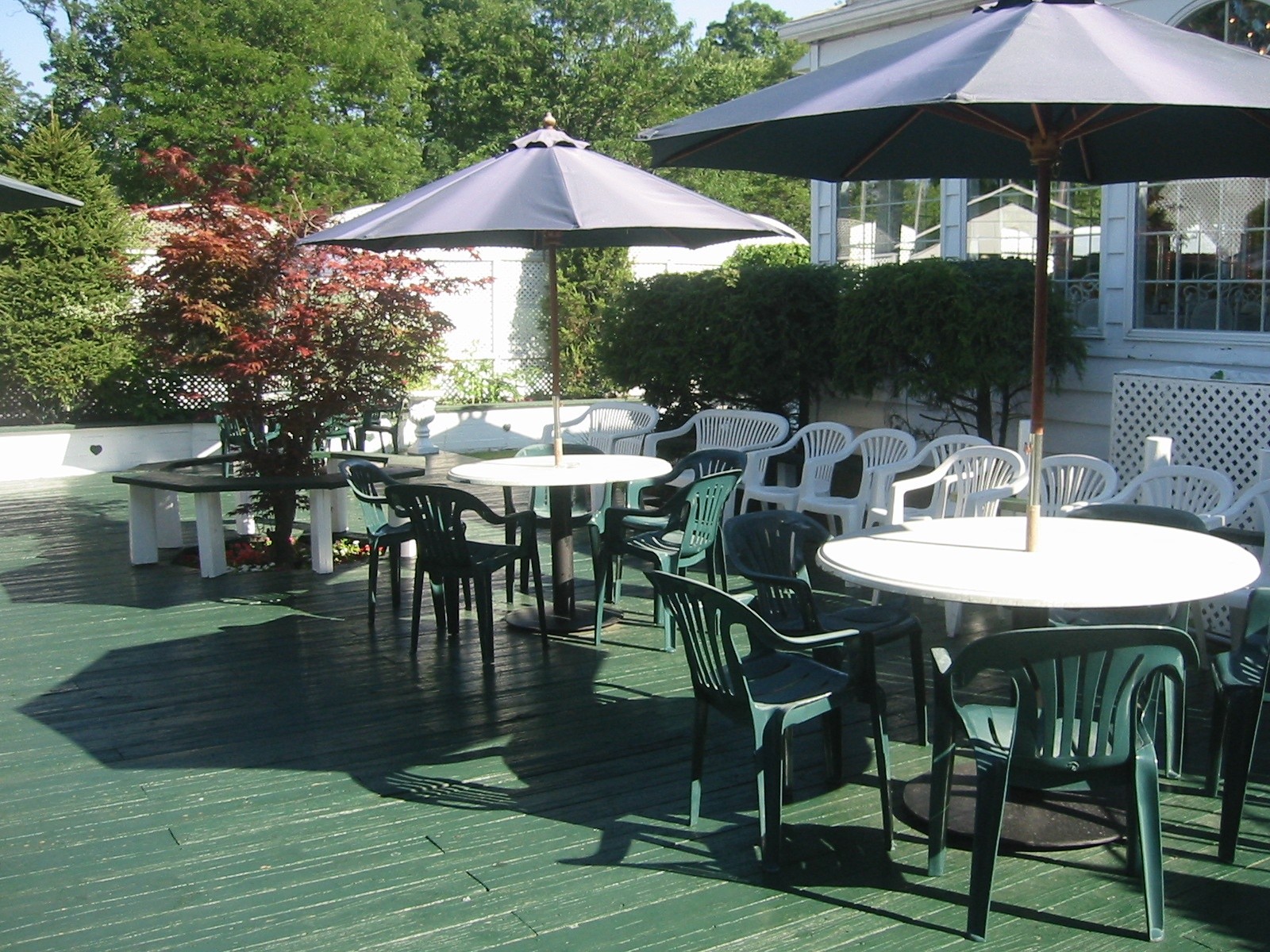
[0,175,84,214]
[293,111,796,466]
[637,1,1270,551]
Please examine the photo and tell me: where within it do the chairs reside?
[339,401,1270,943]
[1067,272,1270,331]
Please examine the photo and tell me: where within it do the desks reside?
[446,454,673,632]
[814,516,1260,850]
